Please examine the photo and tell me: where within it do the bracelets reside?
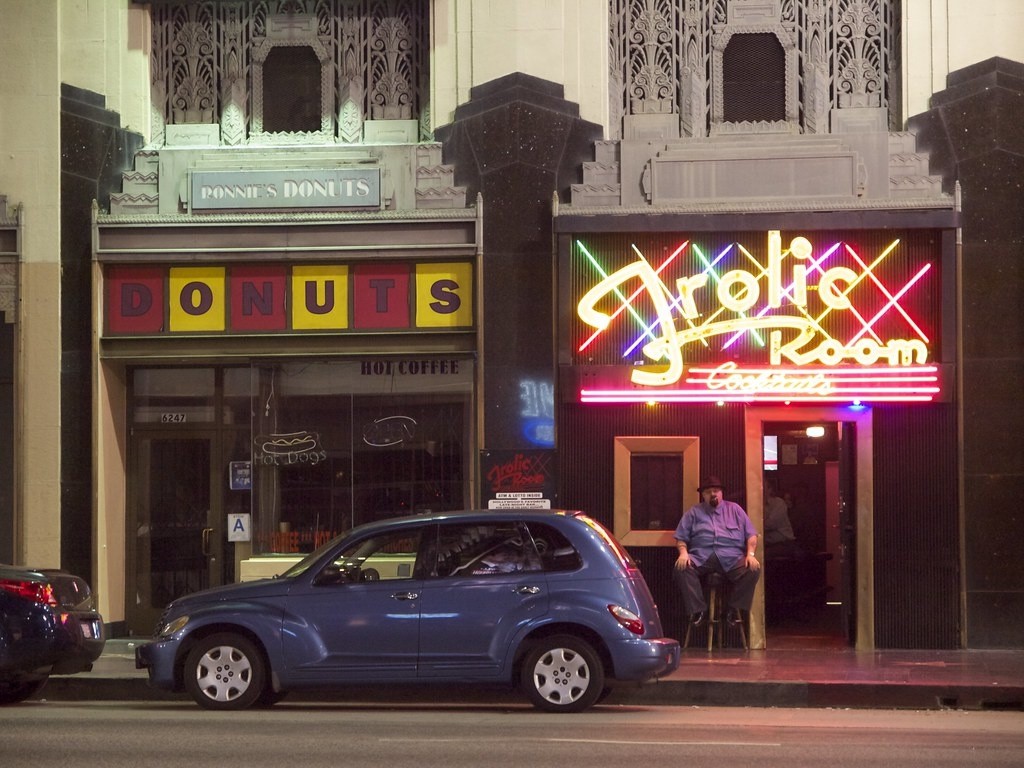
[750,552,755,556]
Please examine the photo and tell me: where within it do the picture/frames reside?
[782,444,799,465]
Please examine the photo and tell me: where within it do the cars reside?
[0,563,105,705]
[134,508,681,714]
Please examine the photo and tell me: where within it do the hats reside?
[697,476,722,491]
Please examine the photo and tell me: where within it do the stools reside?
[685,572,749,651]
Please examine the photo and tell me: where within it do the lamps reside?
[802,440,818,465]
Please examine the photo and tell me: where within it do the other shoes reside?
[721,606,736,626]
[692,609,707,627]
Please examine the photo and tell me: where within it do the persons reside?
[672,478,762,624]
[764,471,797,556]
